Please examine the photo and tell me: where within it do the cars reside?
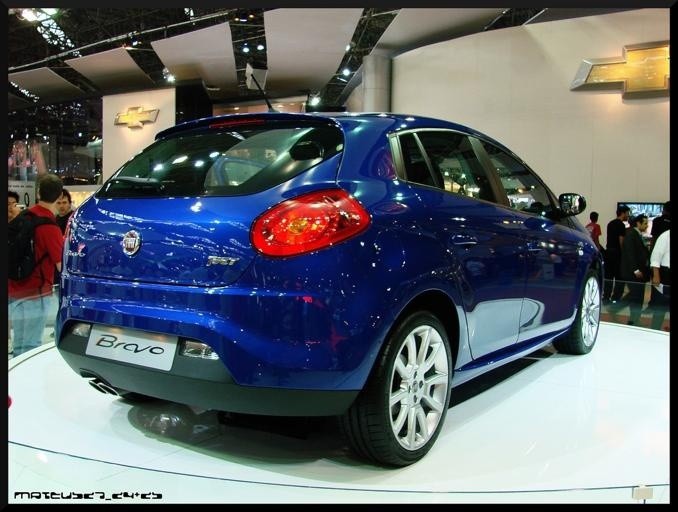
[51,73,607,470]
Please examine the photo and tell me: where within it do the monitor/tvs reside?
[617,202,666,240]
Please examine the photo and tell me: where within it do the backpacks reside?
[7,209,59,281]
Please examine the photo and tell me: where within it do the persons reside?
[586,201,670,331]
[8,174,65,358]
[50,189,73,338]
[8,191,23,355]
